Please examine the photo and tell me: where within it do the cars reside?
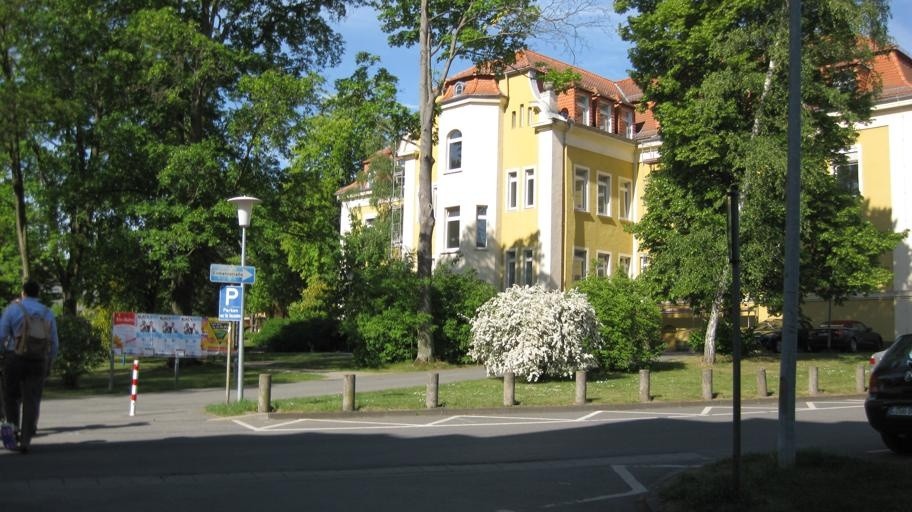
[806,319,883,352]
[748,318,813,353]
[863,331,912,458]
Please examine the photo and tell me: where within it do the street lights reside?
[225,194,262,404]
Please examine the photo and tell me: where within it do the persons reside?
[138,318,199,335]
[0,294,43,439]
[0,279,59,452]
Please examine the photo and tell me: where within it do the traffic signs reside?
[208,262,258,285]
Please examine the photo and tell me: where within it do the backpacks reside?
[13,301,53,368]
